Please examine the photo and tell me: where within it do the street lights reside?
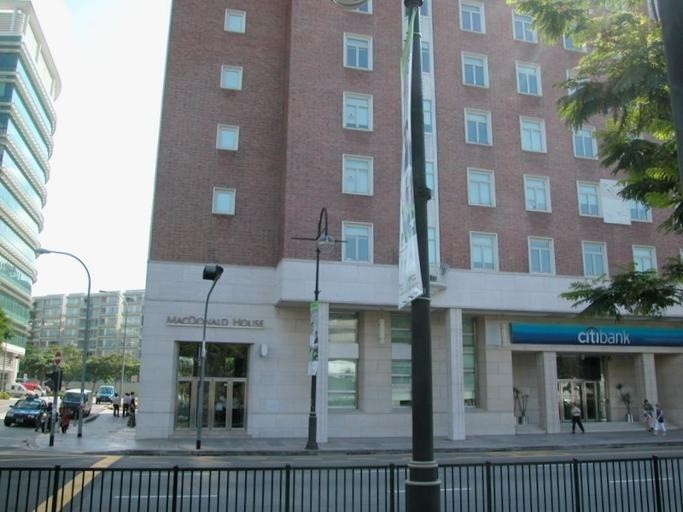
[289,208,348,451]
[97,287,129,405]
[194,263,223,449]
[32,247,92,437]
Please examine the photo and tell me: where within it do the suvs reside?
[95,384,117,405]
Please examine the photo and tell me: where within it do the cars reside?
[3,393,51,427]
[9,382,52,399]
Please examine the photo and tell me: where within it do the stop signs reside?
[53,352,61,363]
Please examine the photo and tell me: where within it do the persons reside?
[571,402,584,434]
[640,399,654,431]
[112,391,136,427]
[652,403,666,436]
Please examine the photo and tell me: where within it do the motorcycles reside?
[35,403,73,432]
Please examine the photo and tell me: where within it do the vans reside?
[58,388,94,419]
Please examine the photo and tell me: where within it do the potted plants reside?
[513,387,529,425]
[616,384,634,423]
